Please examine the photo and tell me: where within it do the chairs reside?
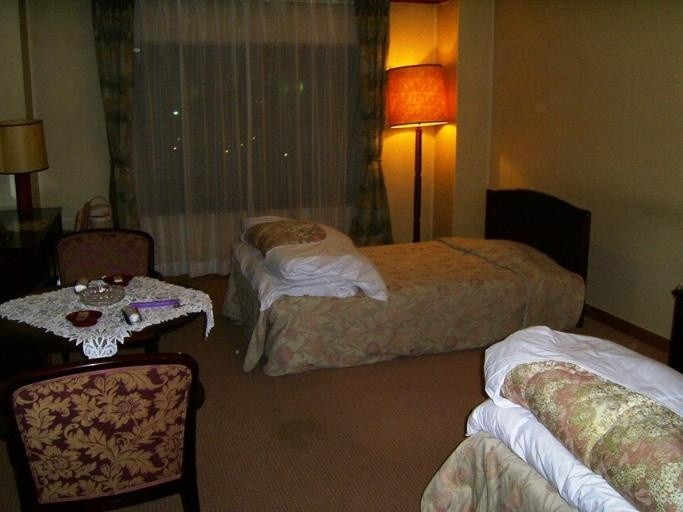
[57,228,160,354]
[2,351,204,511]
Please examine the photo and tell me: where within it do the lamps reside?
[386,63,449,242]
[0,120,50,232]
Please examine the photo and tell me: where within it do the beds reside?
[232,186,592,374]
[467,286,683,512]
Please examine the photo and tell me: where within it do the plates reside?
[80,286,126,306]
[103,274,133,286]
[66,310,103,327]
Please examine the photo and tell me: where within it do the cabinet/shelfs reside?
[0,206,65,368]
